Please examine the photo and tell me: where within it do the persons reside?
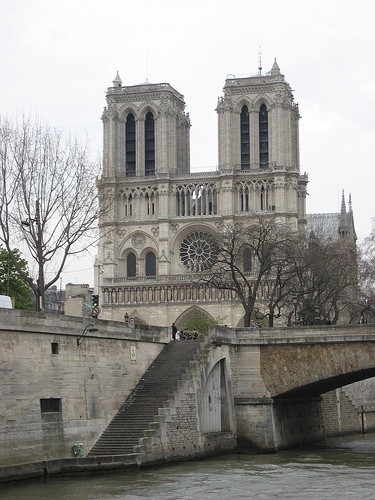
[250,319,256,327]
[175,328,199,340]
[91,302,102,318]
[171,322,177,340]
